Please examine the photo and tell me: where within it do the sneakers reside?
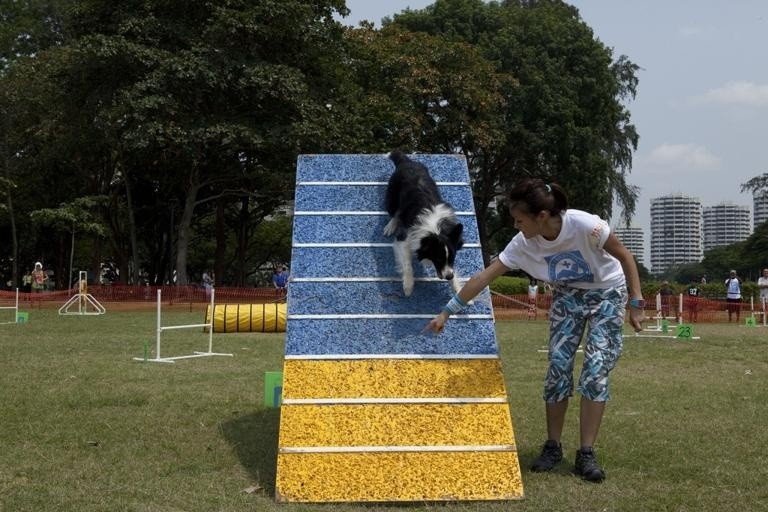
[527,435,567,475]
[571,446,610,486]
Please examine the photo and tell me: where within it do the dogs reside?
[382,149,475,306]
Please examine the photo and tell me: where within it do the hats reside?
[729,268,738,275]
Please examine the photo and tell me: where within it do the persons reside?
[202,269,216,302]
[757,268,768,323]
[725,270,743,323]
[659,273,707,323]
[528,277,539,304]
[30,261,48,309]
[7,280,14,292]
[420,177,645,483]
[22,270,32,301]
[78,274,87,312]
[143,286,152,302]
[272,264,288,289]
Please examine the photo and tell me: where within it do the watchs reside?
[630,299,646,308]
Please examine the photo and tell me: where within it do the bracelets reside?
[444,294,465,315]
